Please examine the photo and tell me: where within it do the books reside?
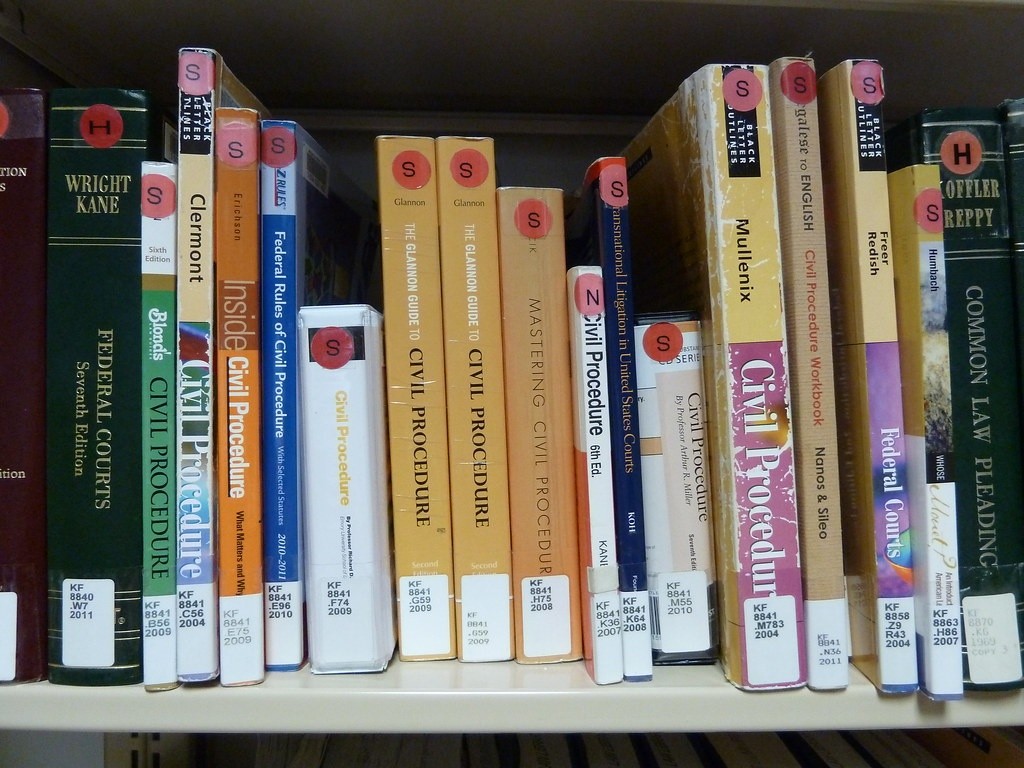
[0,44,1024,701]
[256,729,940,768]
